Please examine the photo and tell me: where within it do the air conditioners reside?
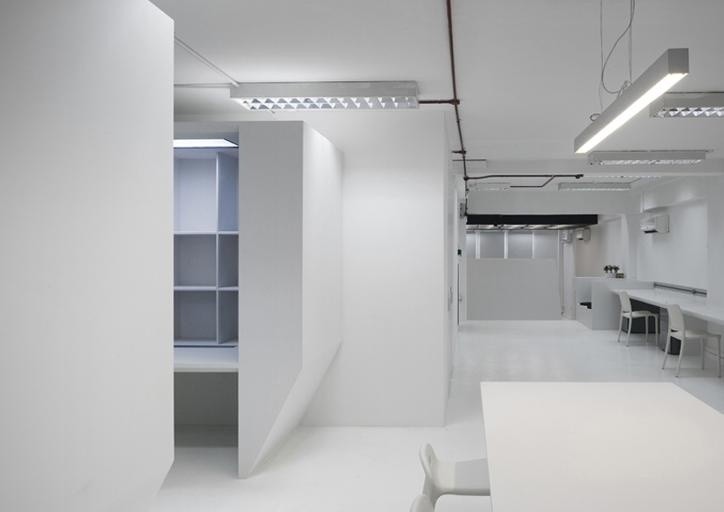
[640,215,668,234]
[560,229,591,244]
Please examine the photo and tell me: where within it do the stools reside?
[409,492,436,511]
[418,441,489,502]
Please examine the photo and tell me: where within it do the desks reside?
[613,288,722,357]
[477,380,724,512]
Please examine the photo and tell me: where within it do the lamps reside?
[228,81,419,112]
[648,91,722,118]
[557,182,630,191]
[588,151,709,166]
[572,0,689,154]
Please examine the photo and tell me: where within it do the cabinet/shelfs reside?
[172,122,239,348]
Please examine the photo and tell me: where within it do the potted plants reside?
[604,265,620,278]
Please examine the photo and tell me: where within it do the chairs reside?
[618,291,658,345]
[661,303,721,377]
